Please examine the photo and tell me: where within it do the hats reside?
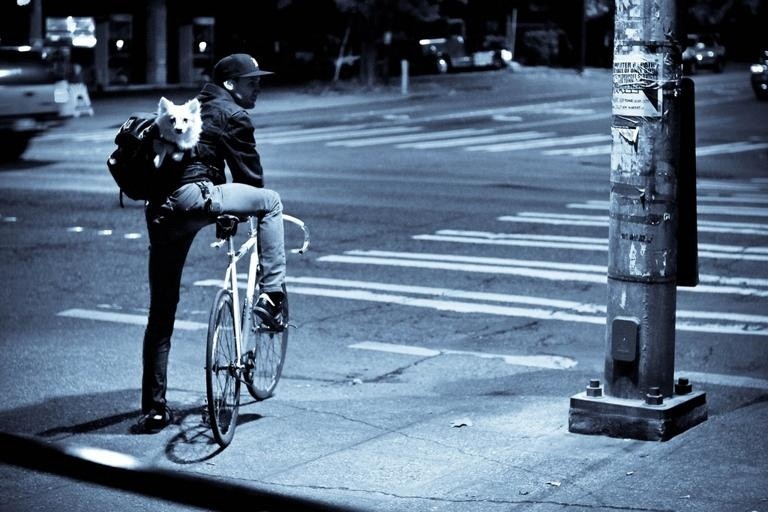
[212,54,276,81]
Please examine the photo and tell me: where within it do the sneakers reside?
[144,407,178,435]
[252,294,289,332]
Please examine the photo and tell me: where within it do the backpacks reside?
[106,95,222,210]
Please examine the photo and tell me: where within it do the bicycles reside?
[204,214,311,447]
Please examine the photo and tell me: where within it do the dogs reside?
[153,96,204,151]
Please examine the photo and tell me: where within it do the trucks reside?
[419,18,510,76]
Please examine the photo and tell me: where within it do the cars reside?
[682,35,724,75]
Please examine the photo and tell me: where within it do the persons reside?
[141,54,288,434]
